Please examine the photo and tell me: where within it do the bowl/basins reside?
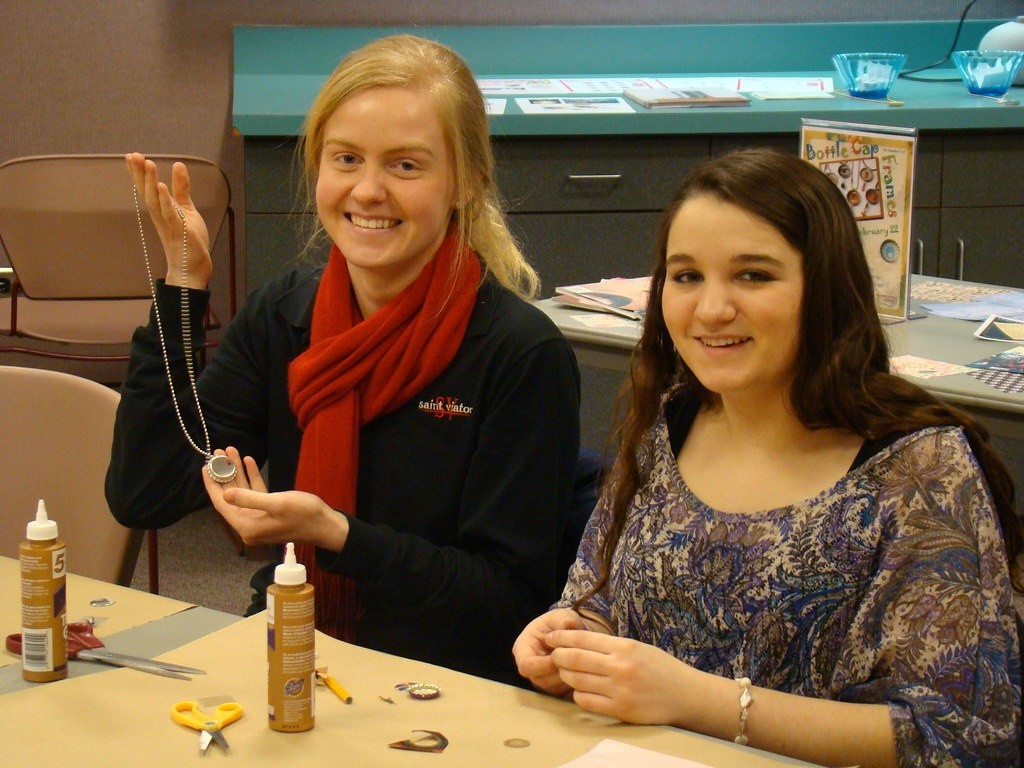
[832,52,908,100]
[950,49,1024,97]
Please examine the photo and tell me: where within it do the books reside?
[551,275,652,320]
[622,88,752,108]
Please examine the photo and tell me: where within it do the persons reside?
[104,32,582,690]
[512,149,1024,768]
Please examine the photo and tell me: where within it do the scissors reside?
[5,620,206,682]
[171,698,244,751]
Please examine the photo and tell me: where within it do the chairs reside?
[0,366,160,595]
[0,153,237,380]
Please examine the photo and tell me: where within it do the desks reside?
[538,263,1024,517]
[0,558,827,768]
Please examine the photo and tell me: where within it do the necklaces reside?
[133,182,237,483]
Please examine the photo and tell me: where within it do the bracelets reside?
[733,677,753,745]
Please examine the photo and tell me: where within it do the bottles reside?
[266,541,317,733]
[19,497,70,684]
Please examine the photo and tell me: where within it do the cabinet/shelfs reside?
[242,126,1024,297]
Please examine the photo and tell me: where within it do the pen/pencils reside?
[320,673,352,704]
